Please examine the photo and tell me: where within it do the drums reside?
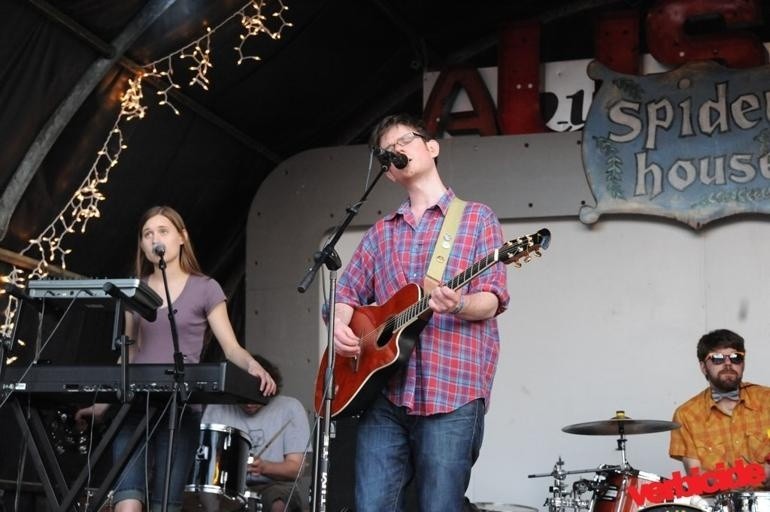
[636,504,706,512]
[242,491,263,511]
[184,424,252,512]
[591,465,688,512]
[714,492,770,512]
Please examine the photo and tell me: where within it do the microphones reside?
[152,242,166,255]
[373,146,408,169]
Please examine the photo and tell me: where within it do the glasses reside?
[705,352,744,365]
[385,132,425,151]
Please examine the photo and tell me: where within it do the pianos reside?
[28,279,162,312]
[1,362,266,405]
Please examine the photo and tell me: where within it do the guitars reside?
[315,228,551,421]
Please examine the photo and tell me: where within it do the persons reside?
[200,353,312,511]
[320,113,513,511]
[668,328,770,492]
[73,204,279,510]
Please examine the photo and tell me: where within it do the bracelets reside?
[447,295,464,315]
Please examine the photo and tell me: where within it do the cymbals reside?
[561,420,681,435]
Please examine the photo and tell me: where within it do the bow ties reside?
[711,389,738,403]
